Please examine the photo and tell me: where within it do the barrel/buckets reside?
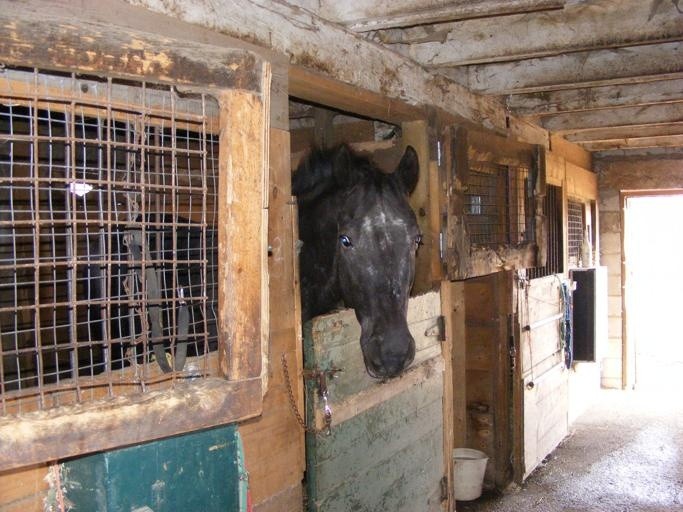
[453,448,489,501]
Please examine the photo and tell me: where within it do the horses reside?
[85,135,425,379]
